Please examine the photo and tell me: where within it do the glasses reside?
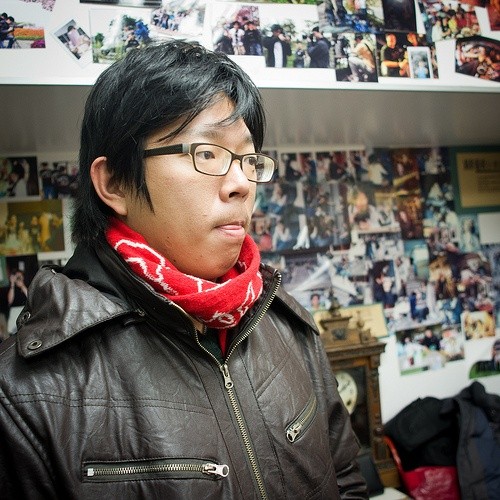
[139,143,279,183]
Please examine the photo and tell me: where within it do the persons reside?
[0,0,500,381]
[0,39,384,500]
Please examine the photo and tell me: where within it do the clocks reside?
[320,297,400,488]
